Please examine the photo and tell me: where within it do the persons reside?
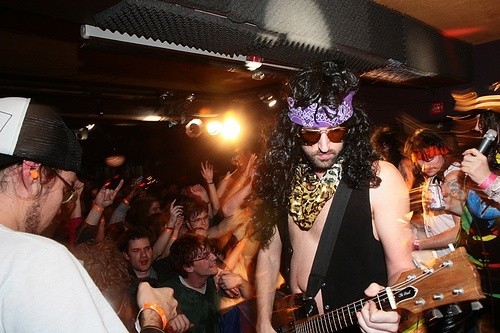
[247,61,414,333]
[0,82,500,333]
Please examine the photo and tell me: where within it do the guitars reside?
[238,244,486,333]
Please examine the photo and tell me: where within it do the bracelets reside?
[206,201,210,204]
[135,303,167,333]
[207,181,214,185]
[92,203,104,212]
[164,224,173,234]
[170,235,178,239]
[123,198,129,206]
[477,172,498,190]
[101,218,105,221]
[414,240,421,250]
[140,324,165,333]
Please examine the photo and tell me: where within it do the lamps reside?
[184,116,203,139]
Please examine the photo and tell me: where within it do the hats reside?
[0,96,82,172]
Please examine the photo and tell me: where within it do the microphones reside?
[465,129,498,179]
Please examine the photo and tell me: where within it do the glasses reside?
[296,125,349,145]
[190,215,209,223]
[194,247,215,262]
[45,165,78,204]
